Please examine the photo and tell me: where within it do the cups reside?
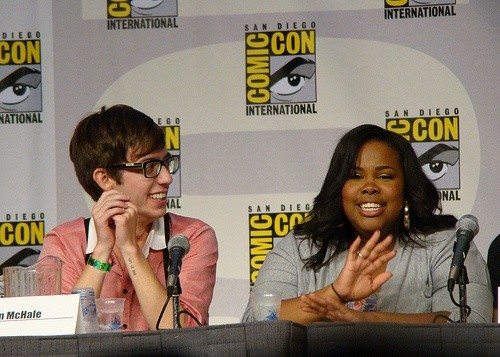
[71,288,99,334]
[95,298,127,332]
[248,291,284,321]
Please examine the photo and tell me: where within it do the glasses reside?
[113,155,181,179]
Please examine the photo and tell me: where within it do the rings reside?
[357,250,368,260]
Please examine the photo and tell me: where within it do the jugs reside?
[2,255,66,298]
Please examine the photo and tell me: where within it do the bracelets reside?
[331,283,348,304]
[85,256,112,272]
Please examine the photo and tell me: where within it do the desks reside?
[0,320,500,357]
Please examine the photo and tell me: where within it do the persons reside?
[487,233,500,322]
[34,104,218,332]
[241,124,493,326]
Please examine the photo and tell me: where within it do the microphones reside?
[167,234,190,296]
[447,214,480,291]
